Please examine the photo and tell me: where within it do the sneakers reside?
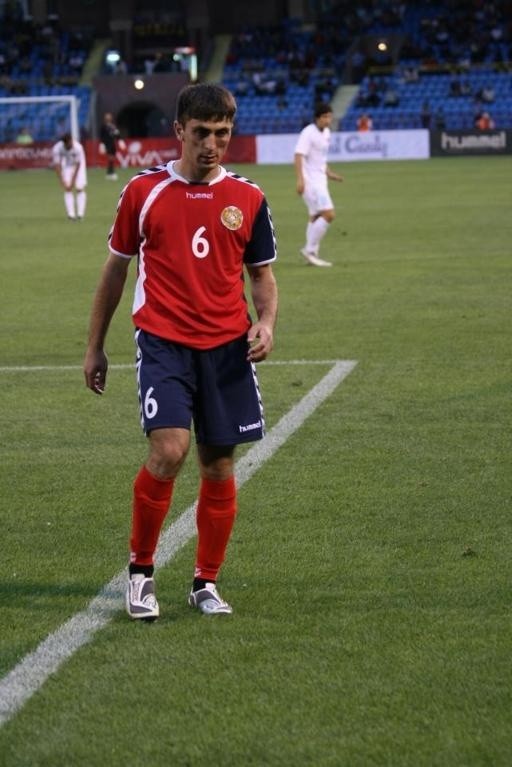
[300,247,330,267]
[124,565,159,618]
[188,582,233,616]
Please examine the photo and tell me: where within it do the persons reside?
[294,102,344,267]
[83,80,278,620]
[98,110,122,179]
[50,133,87,220]
[1,0,511,145]
